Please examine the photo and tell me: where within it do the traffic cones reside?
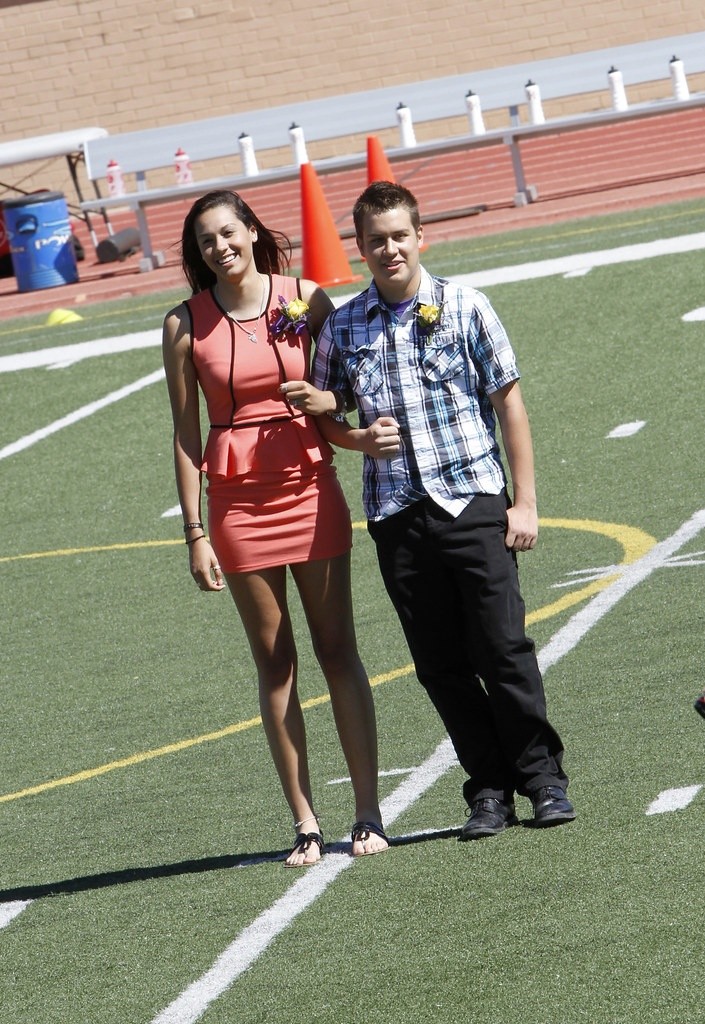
[361,134,429,264]
[300,162,363,286]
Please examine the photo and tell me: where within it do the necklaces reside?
[214,271,266,344]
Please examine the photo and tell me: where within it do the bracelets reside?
[185,535,205,544]
[184,523,204,532]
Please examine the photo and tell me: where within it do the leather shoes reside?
[462,798,518,835]
[531,785,577,823]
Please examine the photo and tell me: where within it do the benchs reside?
[74,28,705,271]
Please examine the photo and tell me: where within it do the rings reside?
[198,582,201,587]
[294,399,297,406]
[212,566,221,572]
[281,385,288,393]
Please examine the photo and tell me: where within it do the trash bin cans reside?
[1,189,80,291]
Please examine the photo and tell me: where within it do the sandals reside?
[351,821,391,856]
[283,829,323,867]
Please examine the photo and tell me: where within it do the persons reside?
[163,188,391,868]
[309,180,578,840]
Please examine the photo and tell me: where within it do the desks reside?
[0,124,111,256]
